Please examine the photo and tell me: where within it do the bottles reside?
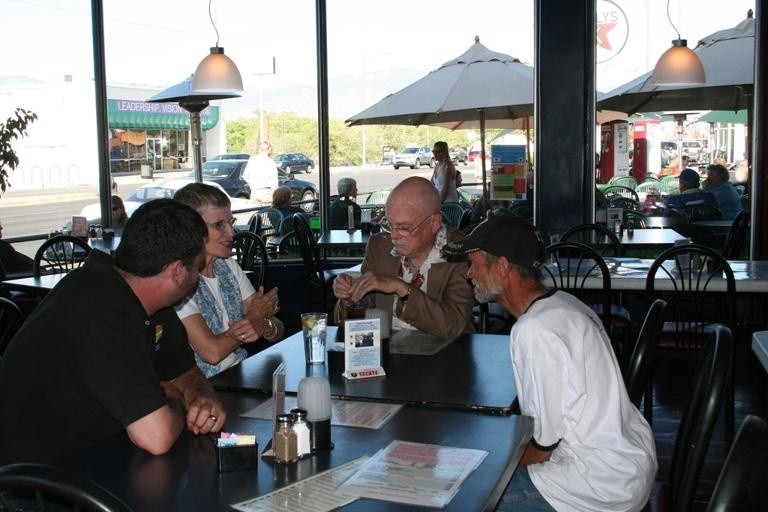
[626,214,632,219]
[62,227,69,246]
[627,216,633,231]
[289,409,312,460]
[95,224,103,241]
[89,224,97,243]
[274,414,298,465]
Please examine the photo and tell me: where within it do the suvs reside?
[450,147,469,166]
[393,144,436,169]
[683,140,709,161]
[661,140,677,166]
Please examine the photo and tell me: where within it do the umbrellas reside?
[345,36,535,220]
[416,109,643,175]
[685,110,748,125]
[595,9,756,202]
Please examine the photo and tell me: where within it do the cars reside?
[276,153,315,175]
[73,178,274,235]
[185,159,320,213]
[48,201,147,259]
[210,153,252,159]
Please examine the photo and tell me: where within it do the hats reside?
[439,210,546,271]
[675,169,700,182]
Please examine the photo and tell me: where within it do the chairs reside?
[509,202,534,220]
[724,210,748,256]
[458,209,473,230]
[621,210,647,229]
[602,186,640,211]
[645,246,738,445]
[441,203,463,228]
[34,236,93,276]
[0,463,132,511]
[558,223,633,365]
[230,231,267,291]
[269,213,297,258]
[243,213,261,270]
[596,174,680,194]
[0,297,26,321]
[641,324,735,512]
[293,213,350,313]
[258,208,283,236]
[534,243,612,336]
[707,416,767,511]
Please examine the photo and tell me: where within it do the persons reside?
[112,196,131,234]
[684,158,690,166]
[440,206,659,511]
[178,151,185,163]
[456,168,471,209]
[703,162,743,222]
[683,149,691,160]
[1,223,46,321]
[270,186,304,215]
[332,177,477,356]
[243,141,278,205]
[327,177,362,233]
[665,168,719,235]
[0,196,227,512]
[431,141,463,202]
[173,183,282,380]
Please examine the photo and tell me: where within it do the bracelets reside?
[271,319,280,339]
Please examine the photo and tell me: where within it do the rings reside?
[208,415,219,422]
[241,332,246,341]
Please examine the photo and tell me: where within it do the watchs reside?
[264,318,274,335]
[401,288,413,302]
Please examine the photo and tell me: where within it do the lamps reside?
[143,78,243,184]
[652,1,707,85]
[192,0,243,94]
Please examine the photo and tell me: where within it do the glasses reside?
[111,205,122,211]
[208,218,237,233]
[432,151,445,154]
[380,213,439,237]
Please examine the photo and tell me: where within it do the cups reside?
[301,312,327,363]
[338,300,366,326]
